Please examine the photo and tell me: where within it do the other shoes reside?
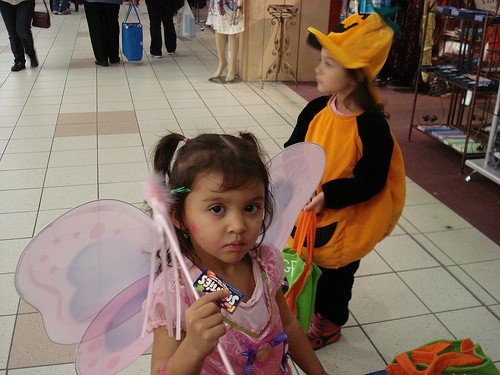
[53,8,70,14]
[110,57,120,63]
[11,62,25,72]
[30,50,38,67]
[95,59,108,66]
[288,312,342,358]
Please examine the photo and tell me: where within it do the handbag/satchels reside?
[32,11,51,28]
[177,0,196,41]
[122,3,142,61]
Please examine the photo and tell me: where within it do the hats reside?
[305,12,394,81]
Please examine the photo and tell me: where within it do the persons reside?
[53,0,72,15]
[0,0,39,72]
[150,129,329,375]
[82,0,140,67]
[283,13,405,353]
[206,0,245,83]
[145,0,183,58]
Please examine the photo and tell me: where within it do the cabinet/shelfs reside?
[408,0,500,186]
